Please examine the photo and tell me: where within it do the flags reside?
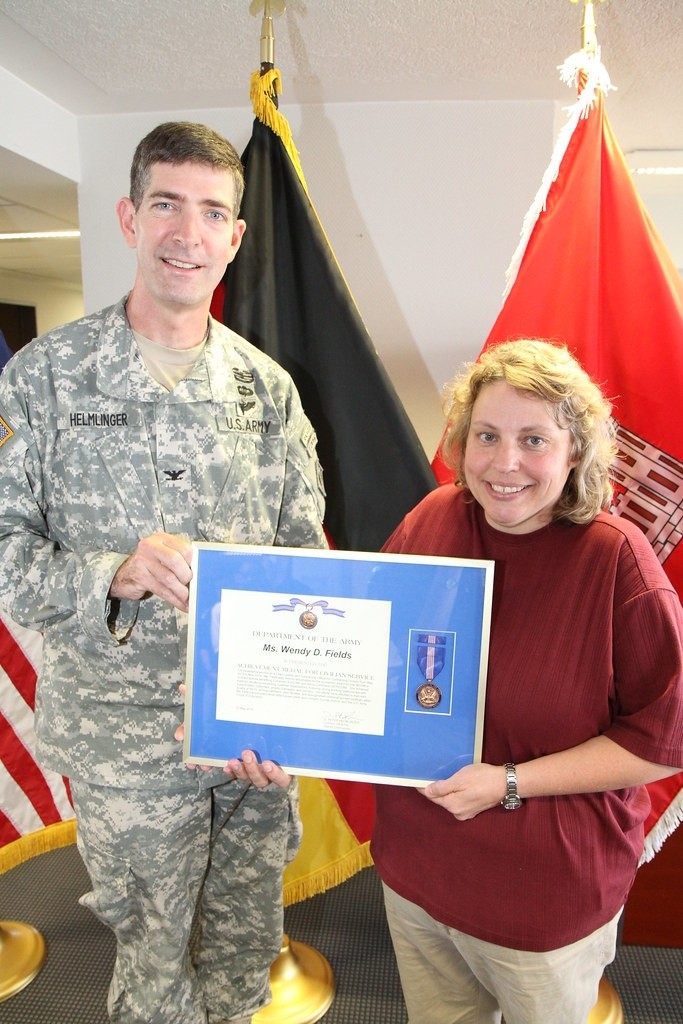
[0,596,76,877]
[207,61,446,907]
[427,31,683,869]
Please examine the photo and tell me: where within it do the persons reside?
[173,335,683,1024]
[0,120,335,1024]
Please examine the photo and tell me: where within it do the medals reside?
[415,682,442,709]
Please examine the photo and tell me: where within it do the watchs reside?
[501,763,524,811]
